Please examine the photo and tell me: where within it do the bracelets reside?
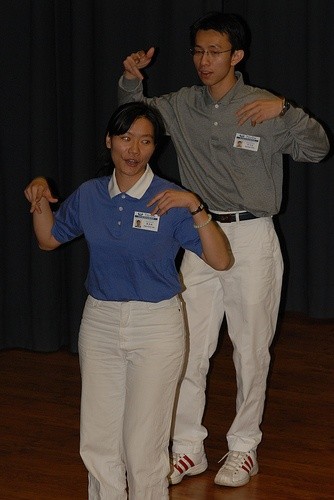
[193,214,212,228]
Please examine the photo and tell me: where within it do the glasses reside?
[191,49,232,58]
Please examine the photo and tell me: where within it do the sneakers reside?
[168,444,209,485]
[213,450,260,488]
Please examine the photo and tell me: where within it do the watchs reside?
[191,203,204,216]
[279,97,290,117]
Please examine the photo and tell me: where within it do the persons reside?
[24,100,230,500]
[119,12,331,489]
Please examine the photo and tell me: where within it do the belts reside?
[206,209,259,224]
